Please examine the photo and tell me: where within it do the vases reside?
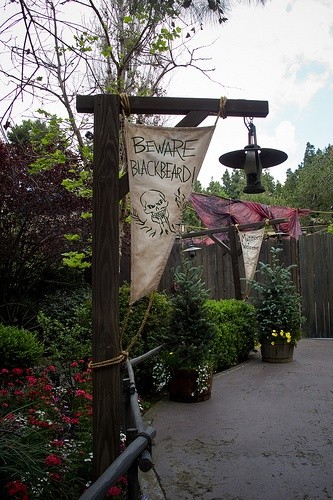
[260,340,294,363]
[165,368,212,403]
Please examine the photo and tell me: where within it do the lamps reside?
[219,116,288,195]
[182,237,202,258]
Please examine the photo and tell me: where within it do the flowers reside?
[150,260,213,397]
[239,246,308,351]
